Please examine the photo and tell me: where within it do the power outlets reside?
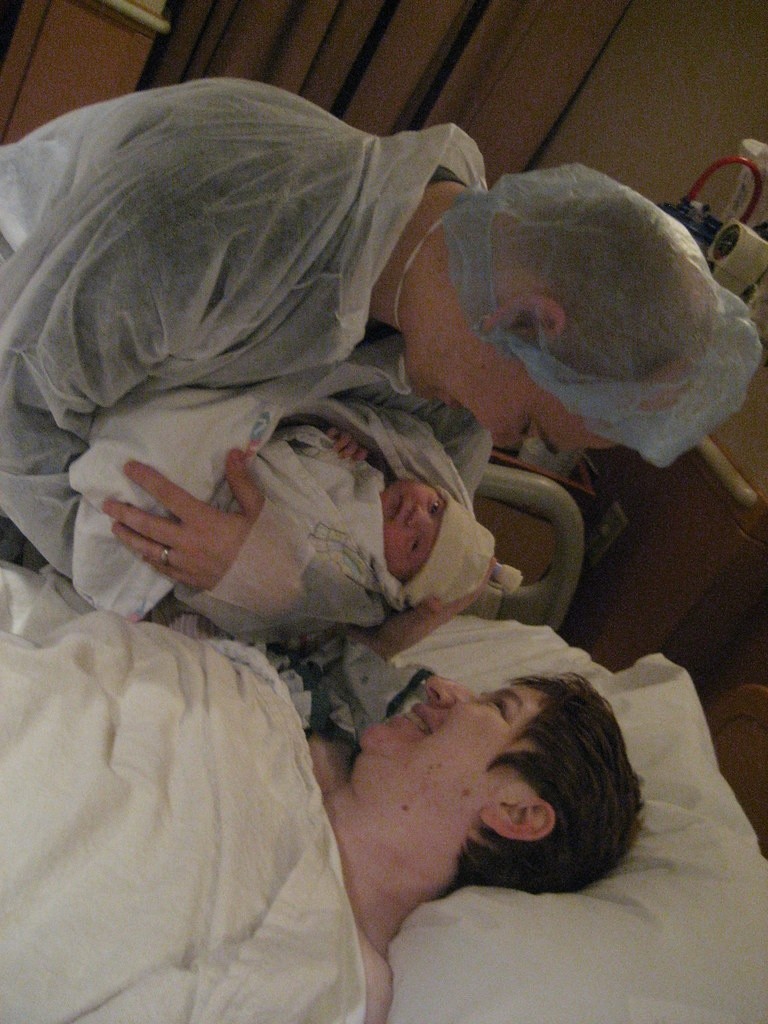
[585,502,629,567]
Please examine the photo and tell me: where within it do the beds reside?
[0,463,767,1024]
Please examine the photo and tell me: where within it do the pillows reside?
[381,652,767,1024]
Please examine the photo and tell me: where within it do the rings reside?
[160,547,171,567]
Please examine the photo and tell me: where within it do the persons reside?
[66,383,523,635]
[1,79,762,644]
[281,555,645,1024]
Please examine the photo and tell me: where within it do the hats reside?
[401,489,526,606]
